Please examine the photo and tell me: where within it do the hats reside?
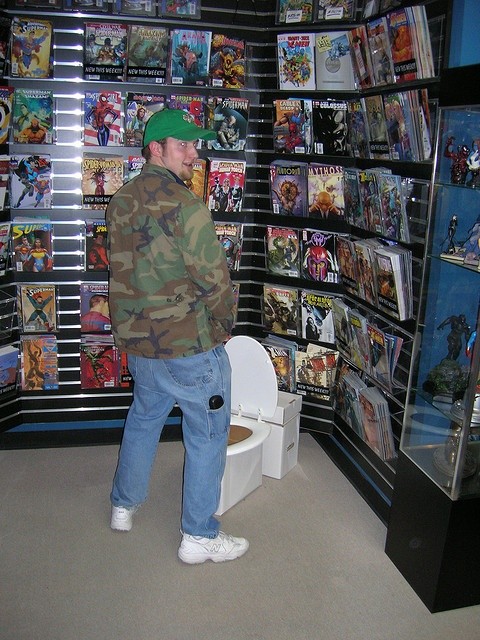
[143,108,217,146]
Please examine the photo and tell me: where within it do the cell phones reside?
[208,396,224,408]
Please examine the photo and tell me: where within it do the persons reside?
[465,323,480,392]
[466,138,480,188]
[104,108,249,563]
[435,310,472,361]
[458,214,480,260]
[439,213,459,256]
[444,136,471,187]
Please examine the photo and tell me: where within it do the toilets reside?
[214,335,302,515]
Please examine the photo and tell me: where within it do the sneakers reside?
[110,505,139,531]
[178,529,249,564]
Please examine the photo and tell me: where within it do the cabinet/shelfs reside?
[385,64,479,615]
[1,1,456,528]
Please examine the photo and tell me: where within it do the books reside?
[205,156,246,214]
[8,152,52,210]
[62,0,109,13]
[0,86,14,146]
[83,21,127,84]
[16,284,56,333]
[123,91,167,148]
[170,27,212,86]
[267,158,416,245]
[275,4,437,91]
[264,224,415,322]
[274,0,402,26]
[209,34,247,90]
[229,283,240,330]
[180,158,207,202]
[80,334,130,391]
[79,282,112,334]
[9,220,55,274]
[260,282,405,396]
[158,0,202,21]
[210,220,244,273]
[204,95,251,152]
[259,334,400,464]
[8,0,62,12]
[126,24,169,84]
[269,86,434,164]
[112,0,157,18]
[10,88,54,145]
[0,335,59,391]
[0,221,11,277]
[84,218,111,272]
[128,155,147,181]
[9,16,55,79]
[81,152,124,211]
[0,155,10,212]
[82,89,122,147]
[168,92,207,150]
